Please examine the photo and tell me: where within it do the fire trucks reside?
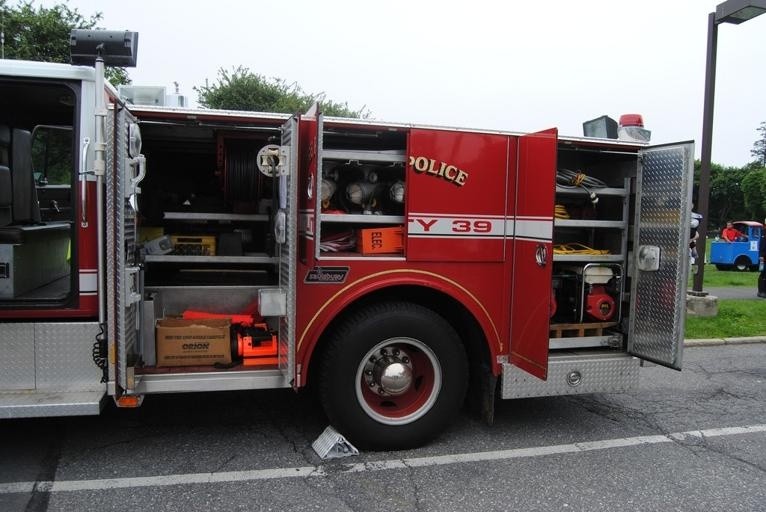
[0,29,702,452]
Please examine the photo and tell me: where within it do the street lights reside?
[693,0,766,292]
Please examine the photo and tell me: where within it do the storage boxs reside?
[155,318,233,367]
[583,115,618,139]
[170,236,216,256]
[357,226,404,254]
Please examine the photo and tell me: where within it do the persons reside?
[721,220,750,244]
[757,217,766,297]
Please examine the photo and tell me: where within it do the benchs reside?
[0,220,71,301]
